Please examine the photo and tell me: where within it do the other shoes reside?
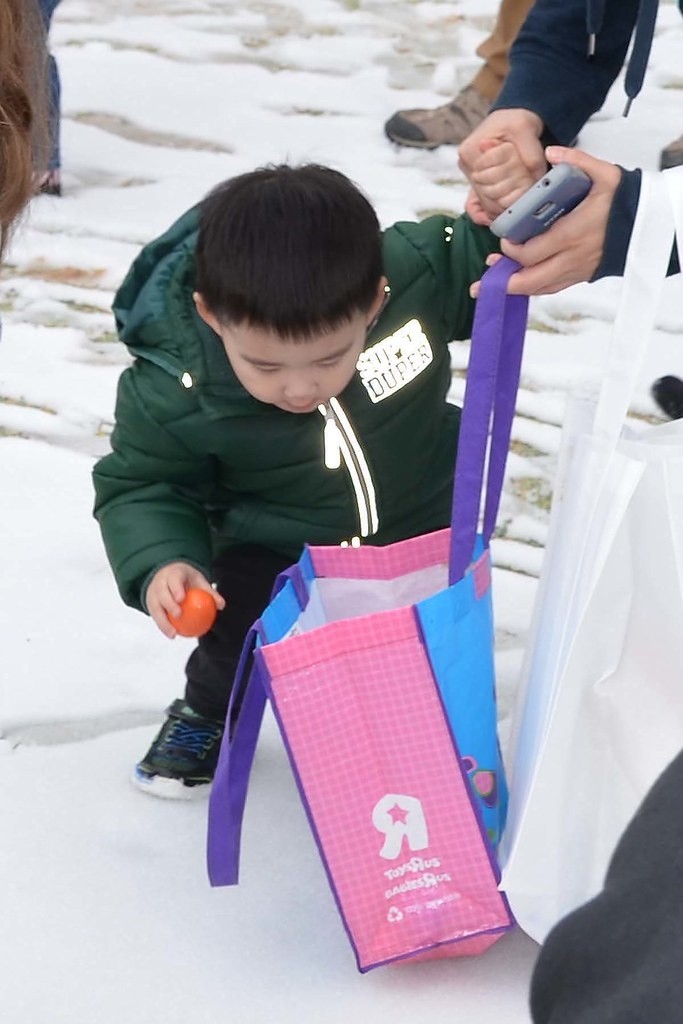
[653,375,683,419]
[385,87,490,148]
[32,167,63,196]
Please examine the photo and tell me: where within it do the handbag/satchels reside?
[205,259,529,973]
[500,175,683,947]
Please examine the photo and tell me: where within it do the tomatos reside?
[166,587,217,637]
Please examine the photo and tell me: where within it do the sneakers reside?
[133,699,227,799]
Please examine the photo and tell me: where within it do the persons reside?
[91,138,536,791]
[386,0,683,1023]
[0,0,61,274]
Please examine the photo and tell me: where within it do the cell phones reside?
[491,162,591,246]
